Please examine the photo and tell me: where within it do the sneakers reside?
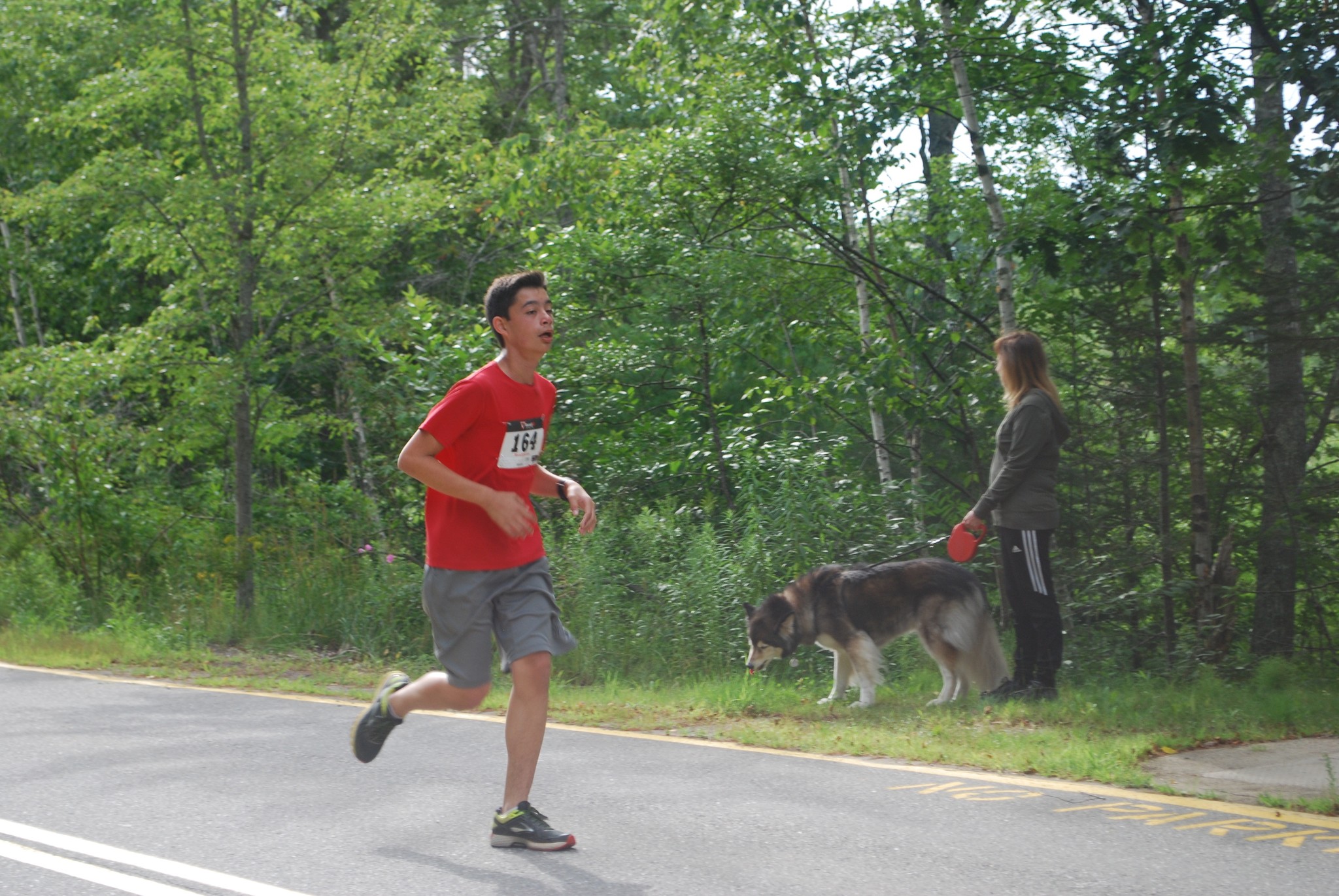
[351,671,410,764]
[489,799,575,853]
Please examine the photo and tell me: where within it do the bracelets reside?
[556,478,574,500]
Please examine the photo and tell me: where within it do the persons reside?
[961,330,1068,704]
[355,271,598,853]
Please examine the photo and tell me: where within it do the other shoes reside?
[979,680,1059,700]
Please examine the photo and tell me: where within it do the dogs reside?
[744,558,1011,707]
